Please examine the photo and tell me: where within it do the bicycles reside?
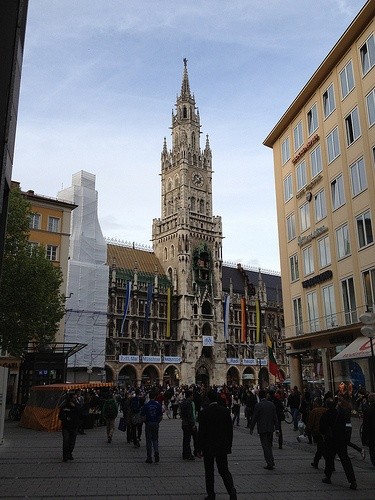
[283,405,293,424]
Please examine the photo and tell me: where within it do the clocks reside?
[191,172,204,187]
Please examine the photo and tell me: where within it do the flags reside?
[120,281,132,335]
[241,298,247,342]
[266,332,286,382]
[255,299,261,342]
[142,283,153,335]
[165,286,171,337]
[224,295,230,339]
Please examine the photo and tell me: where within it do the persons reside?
[319,397,358,490]
[57,380,375,469]
[199,389,237,500]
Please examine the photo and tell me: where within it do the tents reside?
[329,335,375,400]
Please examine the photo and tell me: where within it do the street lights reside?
[254,343,265,371]
[87,365,92,383]
[359,304,375,390]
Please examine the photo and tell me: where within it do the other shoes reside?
[204,493,216,500]
[146,457,152,463]
[182,454,195,460]
[134,443,140,448]
[360,449,365,459]
[321,478,331,484]
[278,447,283,449]
[311,463,318,469]
[264,463,275,470]
[349,483,357,489]
[335,455,340,461]
[230,488,237,500]
[136,436,141,441]
[155,456,159,462]
[62,457,73,462]
[307,440,313,444]
[108,436,112,443]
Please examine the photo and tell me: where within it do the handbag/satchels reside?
[118,417,126,432]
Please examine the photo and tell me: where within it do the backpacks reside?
[58,402,76,423]
